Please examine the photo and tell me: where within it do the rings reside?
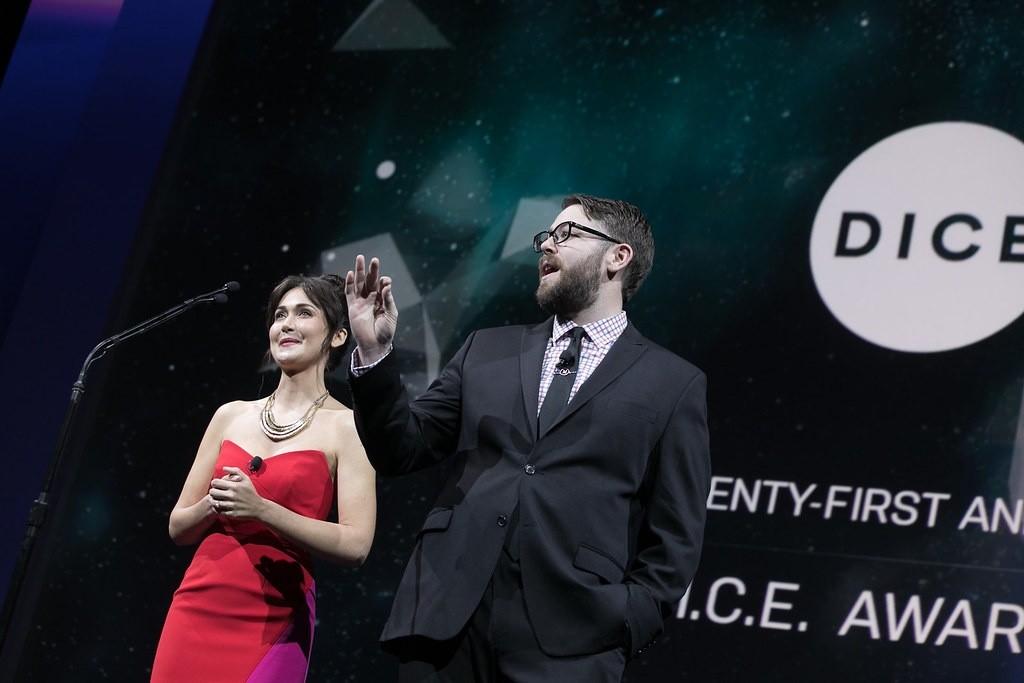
[216,501,220,507]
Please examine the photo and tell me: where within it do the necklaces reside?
[259,390,329,440]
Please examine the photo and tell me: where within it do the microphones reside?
[556,349,572,369]
[249,456,263,470]
[78,281,240,384]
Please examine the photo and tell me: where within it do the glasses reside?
[533,221,622,253]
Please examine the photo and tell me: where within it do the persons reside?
[345,193,712,683]
[150,274,376,683]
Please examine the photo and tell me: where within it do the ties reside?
[503,327,587,562]
